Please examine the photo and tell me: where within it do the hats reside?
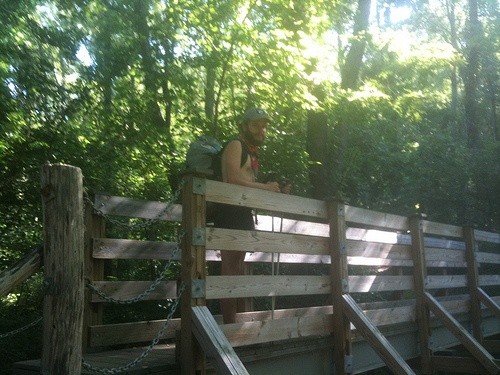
[243,107,272,124]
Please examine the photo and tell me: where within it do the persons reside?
[212,107,292,326]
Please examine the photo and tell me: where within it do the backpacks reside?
[187,127,248,177]
